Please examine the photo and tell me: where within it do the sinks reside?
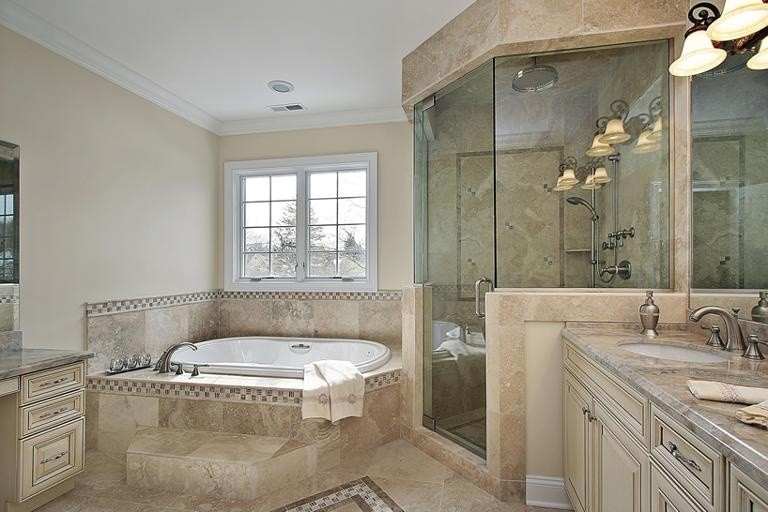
[615,339,734,363]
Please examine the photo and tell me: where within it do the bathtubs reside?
[169,334,393,379]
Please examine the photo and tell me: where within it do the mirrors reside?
[0,142,18,283]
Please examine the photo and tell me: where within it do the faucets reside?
[688,305,768,360]
[153,340,200,376]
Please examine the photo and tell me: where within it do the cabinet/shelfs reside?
[648,399,768,512]
[0,360,93,503]
[564,330,650,512]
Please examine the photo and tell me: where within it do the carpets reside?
[272,477,400,512]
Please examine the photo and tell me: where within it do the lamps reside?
[577,157,612,189]
[584,99,631,158]
[552,156,579,191]
[622,95,663,154]
[668,0,768,77]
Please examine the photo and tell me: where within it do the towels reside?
[436,339,486,388]
[302,360,364,425]
[689,378,768,429]
